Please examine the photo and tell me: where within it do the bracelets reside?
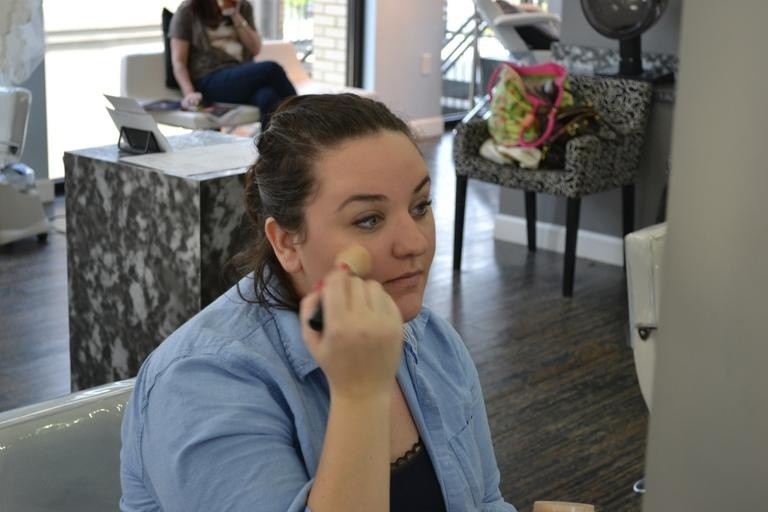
[235,20,247,30]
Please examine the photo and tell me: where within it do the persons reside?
[117,92,521,509]
[168,1,300,137]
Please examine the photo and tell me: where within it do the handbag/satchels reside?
[480,61,600,171]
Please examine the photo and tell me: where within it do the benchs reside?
[116,40,375,144]
[1,88,51,250]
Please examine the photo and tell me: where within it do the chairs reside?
[448,72,656,299]
[444,0,563,71]
[0,376,136,512]
